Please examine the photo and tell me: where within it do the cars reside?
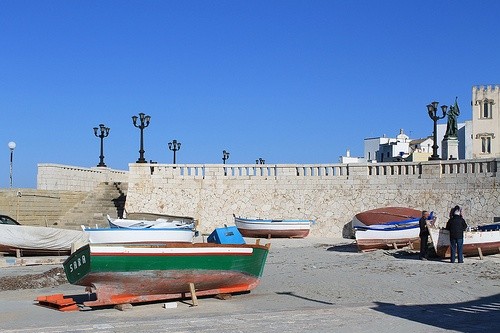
[0,214,22,252]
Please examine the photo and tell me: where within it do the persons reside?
[445,209,467,263]
[419,211,431,260]
[450,205,463,218]
[449,155,453,160]
[446,105,458,137]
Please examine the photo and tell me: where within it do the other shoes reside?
[426,257,431,260]
[419,257,427,260]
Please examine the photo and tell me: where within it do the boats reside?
[351,206,500,260]
[233,213,317,240]
[33,214,272,313]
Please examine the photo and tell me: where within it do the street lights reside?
[131,112,152,163]
[168,139,181,169]
[221,150,230,176]
[7,141,16,188]
[92,123,110,166]
[256,158,268,172]
[426,100,449,160]
